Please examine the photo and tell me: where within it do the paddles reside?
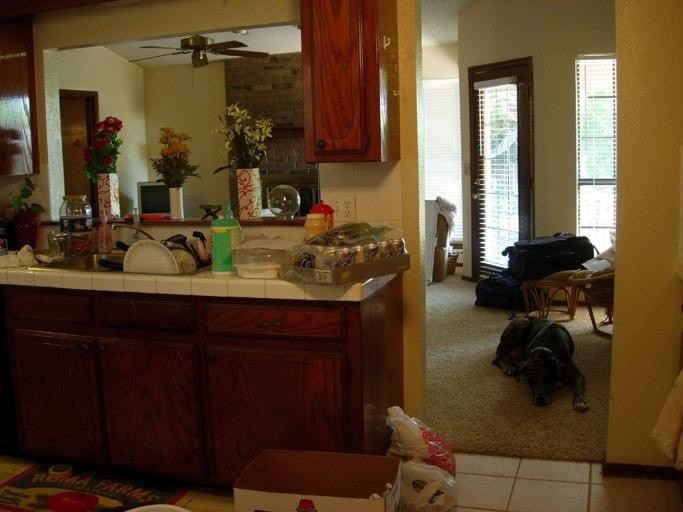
[1,460,189,512]
[426,266,612,463]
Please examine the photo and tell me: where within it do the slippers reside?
[475,274,551,311]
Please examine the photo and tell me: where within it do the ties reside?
[137,182,170,216]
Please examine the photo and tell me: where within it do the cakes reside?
[290,237,406,271]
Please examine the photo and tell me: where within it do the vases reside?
[210,101,273,174]
[74,117,124,184]
[149,124,201,187]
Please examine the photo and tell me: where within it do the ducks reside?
[502,232,599,282]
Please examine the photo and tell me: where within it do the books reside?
[232,448,402,512]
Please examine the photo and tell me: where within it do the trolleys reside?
[130,34,268,67]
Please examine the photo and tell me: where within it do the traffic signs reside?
[326,195,356,222]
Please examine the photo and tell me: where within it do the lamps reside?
[567,246,616,340]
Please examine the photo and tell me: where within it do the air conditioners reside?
[491,316,591,411]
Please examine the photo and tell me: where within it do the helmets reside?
[170,248,196,275]
[122,239,178,275]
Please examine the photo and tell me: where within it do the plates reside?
[0,284,209,494]
[201,273,404,498]
[299,0,390,164]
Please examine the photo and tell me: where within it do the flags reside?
[96,173,121,224]
[169,188,185,220]
[236,167,261,221]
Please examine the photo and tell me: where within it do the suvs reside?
[448,254,459,274]
[433,247,448,282]
[437,213,447,246]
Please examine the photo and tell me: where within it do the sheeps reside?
[106,219,159,241]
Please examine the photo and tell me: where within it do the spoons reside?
[47,492,98,512]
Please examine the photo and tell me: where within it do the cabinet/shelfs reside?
[303,213,325,239]
[309,199,333,230]
[47,228,97,260]
[208,219,240,276]
[58,194,94,235]
